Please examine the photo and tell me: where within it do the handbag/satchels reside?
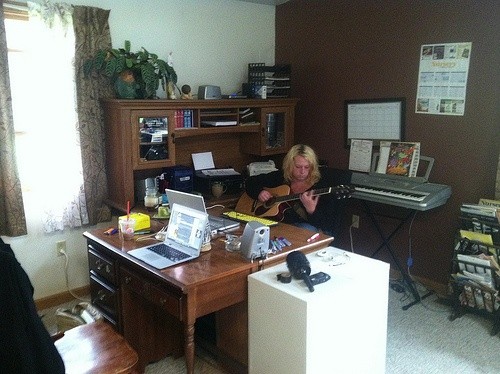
[54,303,103,332]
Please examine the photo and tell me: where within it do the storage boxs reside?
[248,62,292,99]
[117,213,152,233]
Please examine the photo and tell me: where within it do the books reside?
[451,199,500,311]
[202,120,237,125]
[175,110,193,128]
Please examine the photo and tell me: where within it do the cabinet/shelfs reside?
[246,245,390,374]
[99,97,300,220]
[447,212,500,338]
[87,239,186,374]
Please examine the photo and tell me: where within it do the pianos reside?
[324,169,453,310]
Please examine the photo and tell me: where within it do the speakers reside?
[240,220,271,259]
[198,85,221,99]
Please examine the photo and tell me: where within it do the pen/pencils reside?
[135,231,150,234]
[104,227,118,236]
[306,233,319,242]
[270,236,292,255]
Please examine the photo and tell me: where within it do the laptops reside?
[165,189,239,233]
[128,203,209,269]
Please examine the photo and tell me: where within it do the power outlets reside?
[56,240,67,257]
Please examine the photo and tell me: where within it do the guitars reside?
[234,183,356,223]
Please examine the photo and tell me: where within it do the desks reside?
[82,225,259,374]
[207,206,336,271]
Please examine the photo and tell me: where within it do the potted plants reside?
[81,39,178,100]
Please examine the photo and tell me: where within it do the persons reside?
[245,145,330,232]
[0,237,65,374]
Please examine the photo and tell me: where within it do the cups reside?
[119,218,136,240]
[212,181,228,197]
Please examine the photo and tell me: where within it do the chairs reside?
[0,238,139,374]
